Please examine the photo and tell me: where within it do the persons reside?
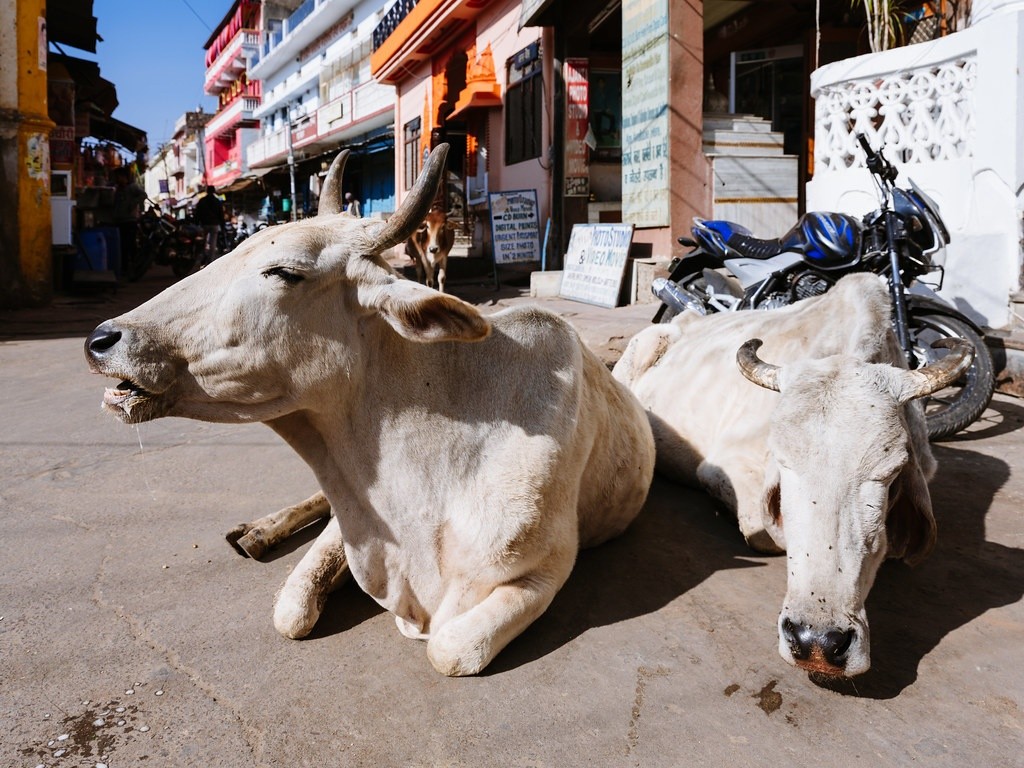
[191,185,228,263]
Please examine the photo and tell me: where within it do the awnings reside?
[171,166,276,210]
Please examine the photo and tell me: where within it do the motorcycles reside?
[648,133,997,442]
[115,192,207,281]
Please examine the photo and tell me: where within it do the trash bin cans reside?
[52,225,123,279]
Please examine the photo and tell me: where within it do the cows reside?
[84,138,975,679]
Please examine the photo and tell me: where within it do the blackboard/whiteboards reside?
[559,223,636,310]
[488,188,543,267]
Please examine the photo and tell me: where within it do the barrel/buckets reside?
[80,231,108,272]
[95,227,122,272]
